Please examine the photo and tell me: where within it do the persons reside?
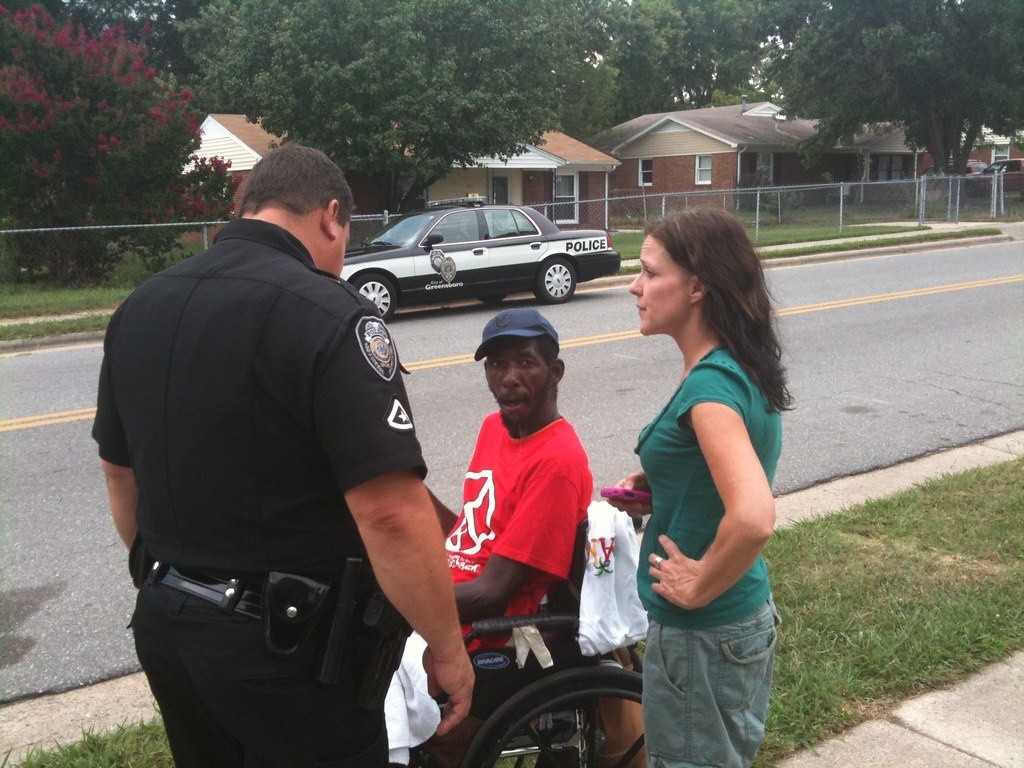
[382,310,593,767]
[92,145,476,768]
[604,209,794,768]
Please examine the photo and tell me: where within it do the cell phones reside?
[600,488,653,504]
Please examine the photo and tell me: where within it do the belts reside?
[150,561,263,622]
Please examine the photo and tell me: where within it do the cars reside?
[339,196,622,323]
[964,158,1024,200]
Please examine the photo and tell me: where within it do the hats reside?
[474,309,559,361]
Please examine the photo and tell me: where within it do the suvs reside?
[915,158,989,190]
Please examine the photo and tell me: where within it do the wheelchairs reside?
[406,515,655,768]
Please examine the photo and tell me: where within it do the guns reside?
[353,588,415,715]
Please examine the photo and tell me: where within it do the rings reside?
[655,557,664,569]
